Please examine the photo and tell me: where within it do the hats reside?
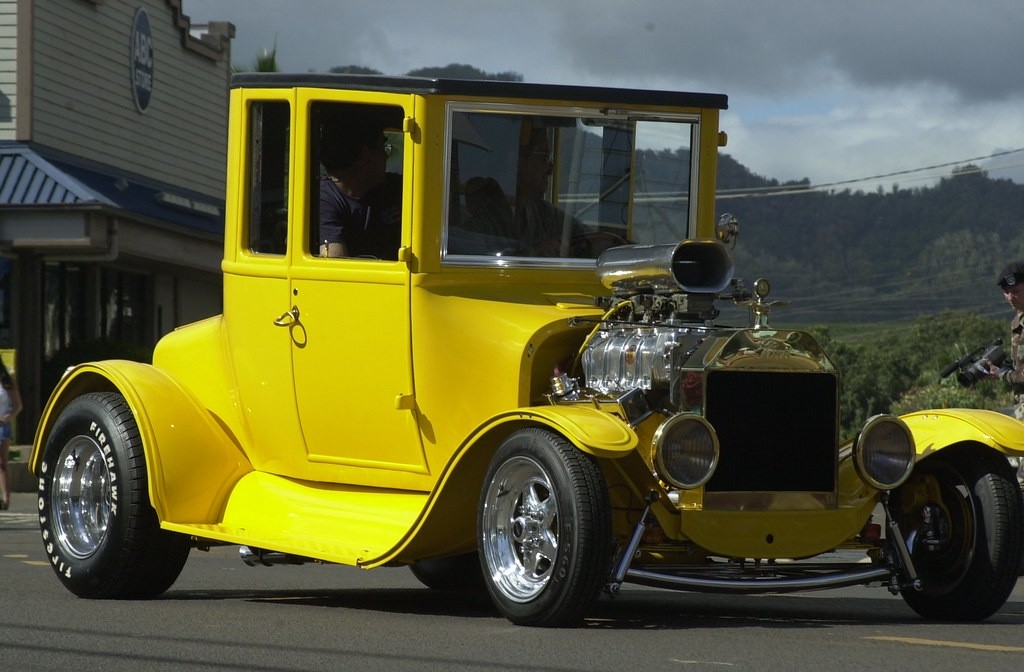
[997,262,1024,289]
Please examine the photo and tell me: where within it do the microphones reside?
[940,348,983,379]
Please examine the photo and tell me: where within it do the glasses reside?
[375,143,393,157]
[527,150,552,162]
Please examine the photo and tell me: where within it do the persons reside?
[313,113,403,262]
[982,262,1024,492]
[450,127,626,258]
[0,356,23,510]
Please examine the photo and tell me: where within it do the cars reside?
[27,71,1024,627]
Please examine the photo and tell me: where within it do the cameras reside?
[958,346,1006,388]
